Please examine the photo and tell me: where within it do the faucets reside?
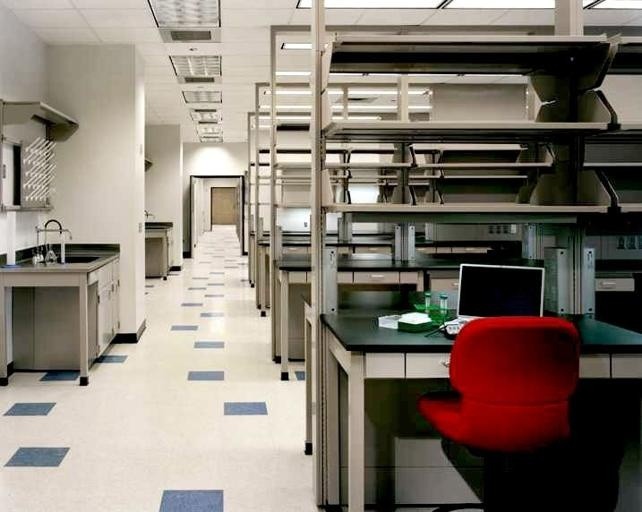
[35,223,73,256]
[43,219,62,253]
[145,209,155,220]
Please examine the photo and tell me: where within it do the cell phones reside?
[444,322,461,336]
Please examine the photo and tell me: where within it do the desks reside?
[249,231,642,512]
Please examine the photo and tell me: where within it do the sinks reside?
[146,225,172,239]
[30,256,102,263]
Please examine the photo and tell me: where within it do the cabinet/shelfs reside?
[247,0,642,224]
[145,229,173,276]
[13,259,122,371]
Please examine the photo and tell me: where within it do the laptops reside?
[439,264,546,332]
